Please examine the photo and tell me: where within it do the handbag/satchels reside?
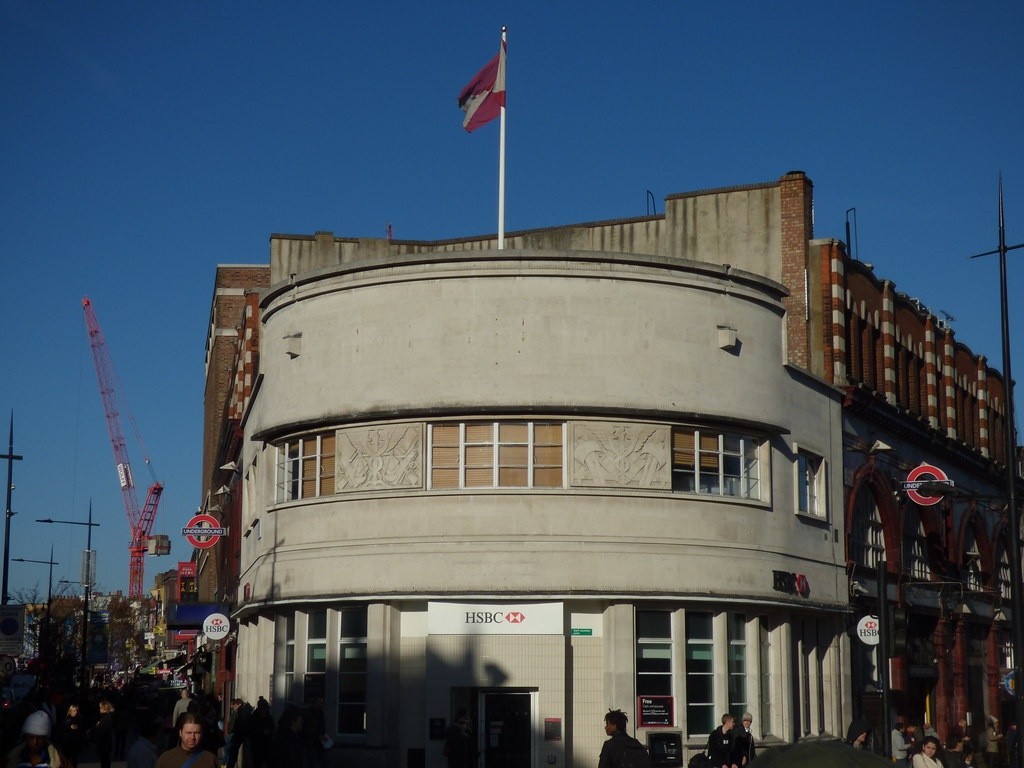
[688,752,712,768]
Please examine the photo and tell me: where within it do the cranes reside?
[80,295,172,669]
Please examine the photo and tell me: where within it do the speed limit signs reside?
[0,656,16,678]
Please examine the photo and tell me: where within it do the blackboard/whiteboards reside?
[637,694,674,727]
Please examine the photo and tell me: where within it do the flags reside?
[456,37,508,134]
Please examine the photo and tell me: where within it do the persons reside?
[170,686,227,756]
[127,720,162,768]
[0,683,148,761]
[598,709,650,768]
[907,736,948,768]
[890,720,941,768]
[4,710,73,768]
[951,719,975,768]
[87,700,116,768]
[844,717,874,752]
[1007,720,1017,768]
[155,711,219,768]
[222,695,326,768]
[984,715,1003,768]
[60,704,83,768]
[709,714,757,768]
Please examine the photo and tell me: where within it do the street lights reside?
[36,519,90,689]
[12,558,52,648]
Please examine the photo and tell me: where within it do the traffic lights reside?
[888,607,912,657]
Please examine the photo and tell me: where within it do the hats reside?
[20,710,52,737]
[989,715,999,724]
[742,713,752,722]
